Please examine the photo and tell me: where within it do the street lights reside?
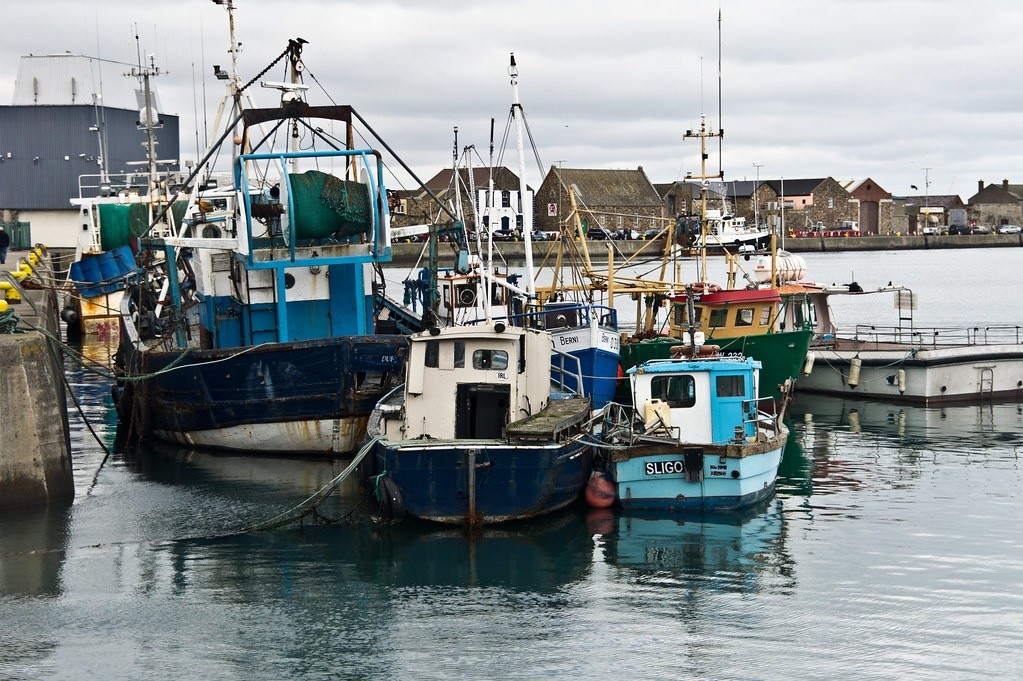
[753,164,765,231]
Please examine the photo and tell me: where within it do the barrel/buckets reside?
[644,398,671,432]
[70,245,139,298]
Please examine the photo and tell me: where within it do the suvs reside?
[948,223,971,235]
[587,228,615,241]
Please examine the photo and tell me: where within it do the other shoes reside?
[1,259,4,264]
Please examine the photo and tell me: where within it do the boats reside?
[62,0,818,523]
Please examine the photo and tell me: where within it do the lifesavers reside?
[669,344,723,363]
[685,283,721,292]
[373,474,404,517]
[111,385,134,424]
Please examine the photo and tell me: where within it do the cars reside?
[637,229,668,241]
[973,226,989,235]
[398,234,451,243]
[999,225,1021,235]
[467,229,563,242]
[612,229,640,241]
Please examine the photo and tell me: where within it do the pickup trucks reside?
[923,225,950,236]
